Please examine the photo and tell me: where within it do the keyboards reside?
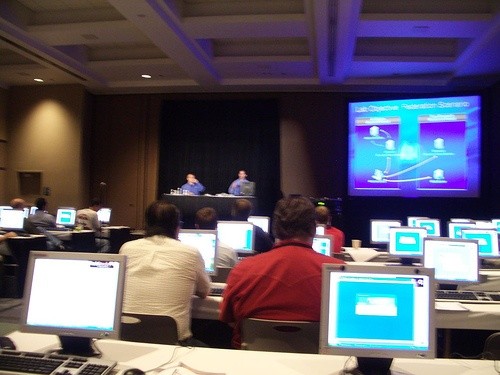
[210,288,224,295]
[0,348,118,375]
[17,234,30,237]
[46,227,66,231]
[434,290,500,302]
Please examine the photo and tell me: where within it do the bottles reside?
[170,188,174,194]
[176,187,180,194]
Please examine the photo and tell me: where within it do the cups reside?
[352,240,362,248]
[174,189,188,195]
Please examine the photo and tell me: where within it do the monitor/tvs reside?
[22,250,126,358]
[176,214,500,290]
[0,205,112,232]
[320,262,436,375]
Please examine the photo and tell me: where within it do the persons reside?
[0,229,17,264]
[181,173,206,195]
[10,198,41,235]
[28,198,56,227]
[232,198,274,255]
[75,198,110,253]
[194,207,238,269]
[228,169,250,196]
[218,194,346,350]
[119,201,210,348]
[313,206,345,253]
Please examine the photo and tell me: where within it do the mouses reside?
[124,368,145,375]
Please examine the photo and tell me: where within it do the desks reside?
[0,224,136,297]
[1,329,500,375]
[193,282,500,331]
[159,194,257,230]
